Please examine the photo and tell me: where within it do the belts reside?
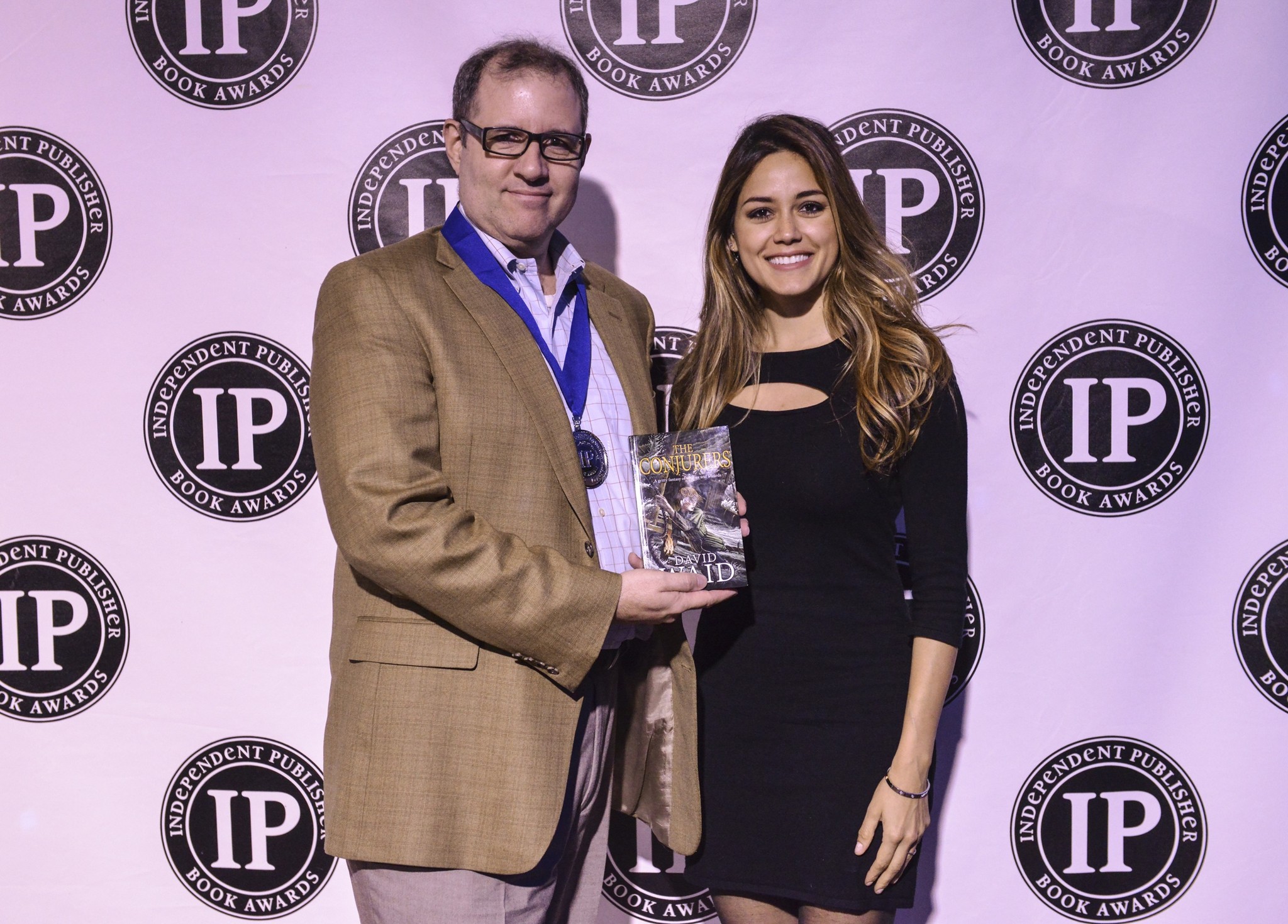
[593,643,631,670]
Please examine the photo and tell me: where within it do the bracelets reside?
[885,767,930,799]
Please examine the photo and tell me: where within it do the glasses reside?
[455,115,585,162]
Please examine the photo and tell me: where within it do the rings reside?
[907,849,916,855]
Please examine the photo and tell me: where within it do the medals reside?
[573,430,609,488]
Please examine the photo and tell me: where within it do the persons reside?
[657,112,968,924]
[309,38,750,924]
